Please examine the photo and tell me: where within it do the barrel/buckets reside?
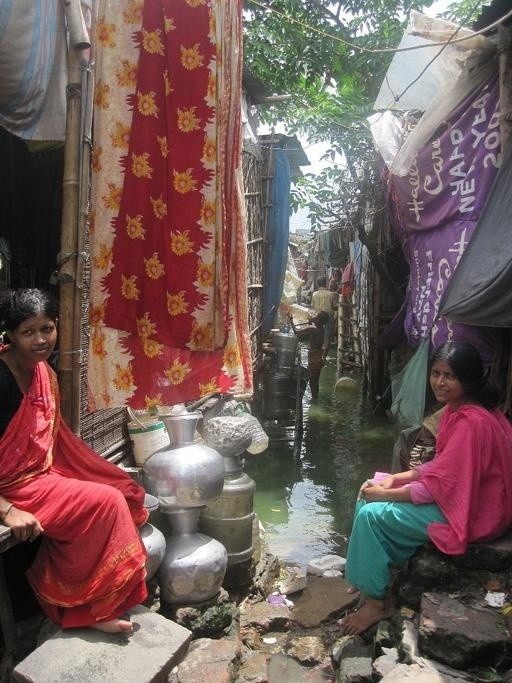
[127,418,170,468]
[234,403,269,455]
[201,454,257,565]
[273,323,297,374]
[287,304,313,322]
[263,368,290,419]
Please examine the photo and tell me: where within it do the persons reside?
[1,285,153,638]
[336,337,512,638]
[328,269,342,292]
[309,276,340,367]
[286,309,329,404]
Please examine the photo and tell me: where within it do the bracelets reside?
[0,502,15,523]
